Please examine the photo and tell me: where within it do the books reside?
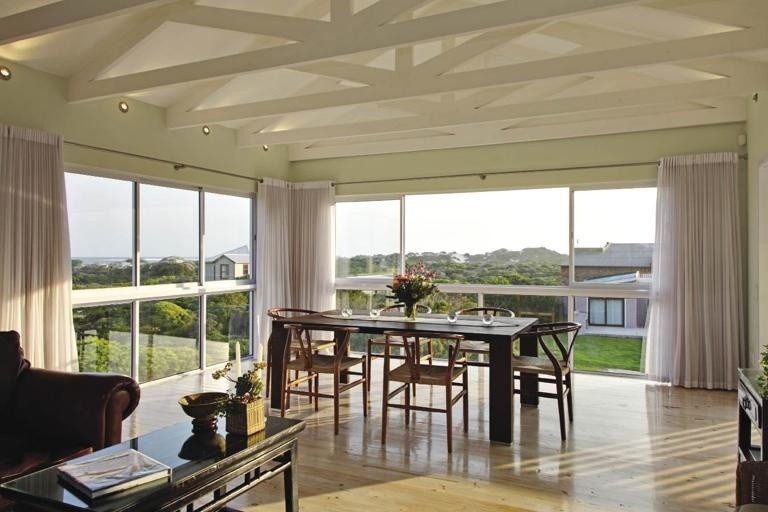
[56,448,173,499]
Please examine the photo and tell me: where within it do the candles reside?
[235,341,242,381]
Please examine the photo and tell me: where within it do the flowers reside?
[384,261,442,321]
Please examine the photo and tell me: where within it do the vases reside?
[404,300,418,322]
[176,391,229,439]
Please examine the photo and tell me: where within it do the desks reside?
[271,309,540,446]
[735,366,767,467]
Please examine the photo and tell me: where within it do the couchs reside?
[0,329,142,485]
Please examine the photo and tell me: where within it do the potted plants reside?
[210,350,278,437]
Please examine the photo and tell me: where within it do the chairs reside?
[265,307,338,397]
[281,324,368,436]
[366,303,433,392]
[449,306,516,367]
[381,329,469,454]
[512,321,583,441]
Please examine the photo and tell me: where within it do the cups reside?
[341,307,353,317]
[446,311,457,324]
[483,313,496,325]
[370,309,380,319]
